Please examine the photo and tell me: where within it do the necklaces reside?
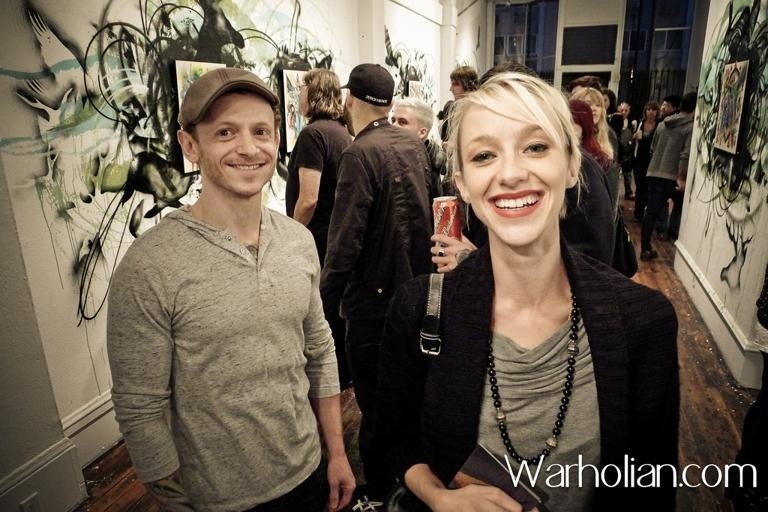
[484,290,587,463]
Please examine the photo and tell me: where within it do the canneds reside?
[431,195,463,267]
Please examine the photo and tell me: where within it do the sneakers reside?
[657,231,672,241]
[633,215,645,222]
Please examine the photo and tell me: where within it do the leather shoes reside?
[628,193,636,200]
[640,248,658,261]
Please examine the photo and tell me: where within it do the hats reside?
[177,67,280,128]
[337,63,394,107]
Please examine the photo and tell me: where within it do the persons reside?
[320,64,446,511]
[380,70,680,511]
[391,64,702,279]
[285,67,352,391]
[108,67,356,511]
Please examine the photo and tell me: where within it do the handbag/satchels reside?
[613,213,638,279]
[347,271,445,512]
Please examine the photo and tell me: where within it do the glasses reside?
[299,84,310,90]
